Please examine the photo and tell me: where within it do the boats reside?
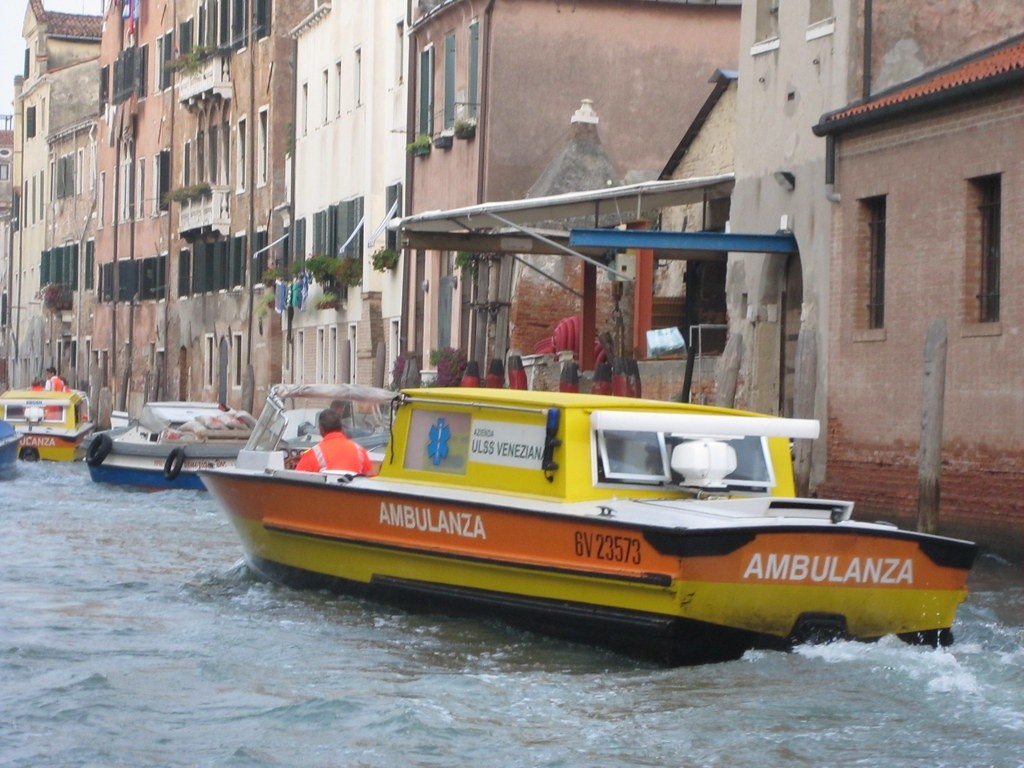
[81,400,290,491]
[194,384,981,670]
[0,388,96,463]
[0,420,24,480]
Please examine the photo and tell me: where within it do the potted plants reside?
[406,133,429,156]
[433,131,452,148]
[454,119,474,139]
[192,51,205,60]
[204,47,215,57]
[368,246,398,273]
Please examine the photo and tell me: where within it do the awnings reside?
[385,172,734,231]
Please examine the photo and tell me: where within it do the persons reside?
[27,367,69,420]
[295,401,377,477]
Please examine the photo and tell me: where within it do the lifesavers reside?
[85,433,113,468]
[161,446,186,481]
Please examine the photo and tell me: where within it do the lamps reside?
[773,171,795,192]
